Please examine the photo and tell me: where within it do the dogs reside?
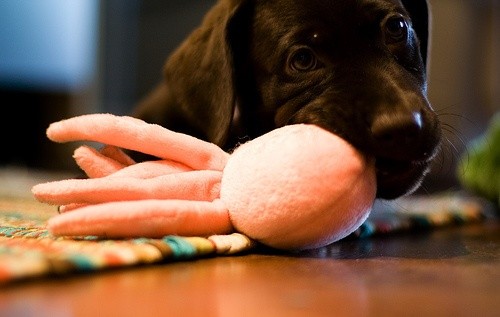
[72,0,494,210]
